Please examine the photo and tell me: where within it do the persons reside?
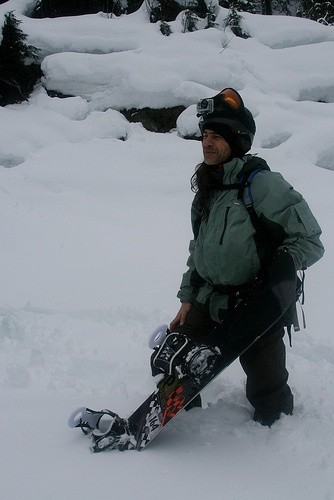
[167,88,326,426]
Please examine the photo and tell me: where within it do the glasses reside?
[217,88,244,110]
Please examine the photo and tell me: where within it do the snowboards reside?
[128,251,297,451]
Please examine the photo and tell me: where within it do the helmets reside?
[197,99,255,154]
